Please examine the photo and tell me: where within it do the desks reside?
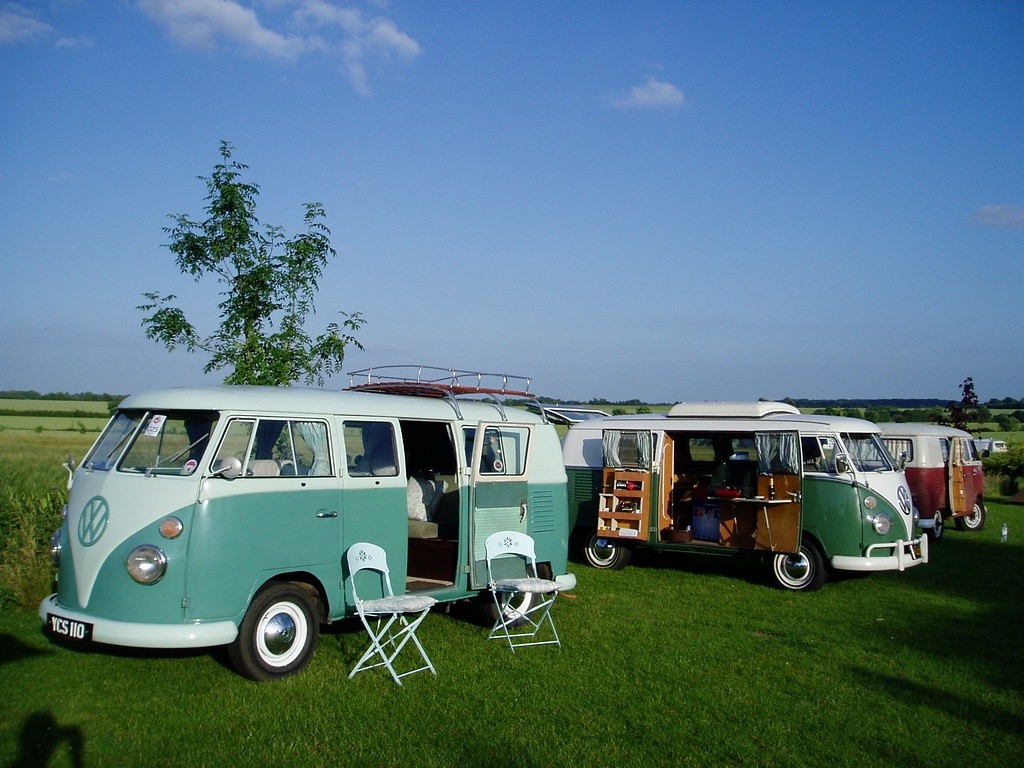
[707,495,792,551]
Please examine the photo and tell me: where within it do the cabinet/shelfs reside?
[597,471,651,540]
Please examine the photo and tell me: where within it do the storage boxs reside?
[426,480,449,521]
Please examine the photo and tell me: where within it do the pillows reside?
[408,476,428,523]
[415,477,435,506]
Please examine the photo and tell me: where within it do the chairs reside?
[347,542,438,685]
[407,475,460,542]
[247,460,309,476]
[804,463,821,471]
[485,531,563,653]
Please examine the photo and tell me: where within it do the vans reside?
[36,364,579,684]
[520,400,931,594]
[830,422,988,532]
